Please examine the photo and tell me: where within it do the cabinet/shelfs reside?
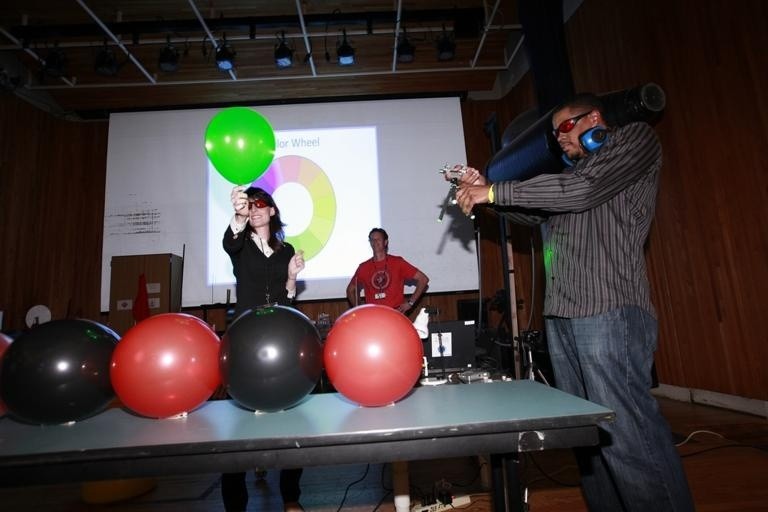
[110,253,183,336]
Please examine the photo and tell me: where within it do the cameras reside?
[522,329,543,346]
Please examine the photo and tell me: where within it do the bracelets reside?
[487,182,495,204]
[407,300,416,307]
[287,278,297,280]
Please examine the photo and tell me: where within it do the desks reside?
[0,376,617,511]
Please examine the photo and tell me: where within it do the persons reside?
[220,186,304,511]
[346,228,429,313]
[455,95,695,510]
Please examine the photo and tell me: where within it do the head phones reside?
[561,126,612,168]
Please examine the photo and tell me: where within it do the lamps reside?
[7,26,461,82]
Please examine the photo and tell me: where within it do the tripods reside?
[523,347,550,386]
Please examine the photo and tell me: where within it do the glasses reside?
[552,111,591,138]
[249,200,268,209]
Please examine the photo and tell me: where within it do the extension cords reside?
[412,495,471,512]
